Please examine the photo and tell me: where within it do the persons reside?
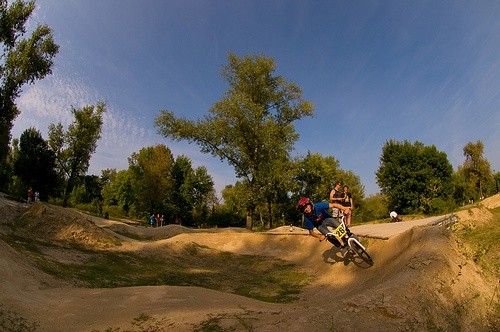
[341,185,354,229]
[27,186,40,203]
[104,212,109,219]
[149,214,164,228]
[388,211,398,223]
[298,197,354,258]
[329,182,345,218]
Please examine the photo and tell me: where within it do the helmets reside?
[298,197,314,216]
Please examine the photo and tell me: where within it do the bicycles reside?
[319,207,374,266]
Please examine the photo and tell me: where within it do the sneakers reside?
[339,248,348,256]
